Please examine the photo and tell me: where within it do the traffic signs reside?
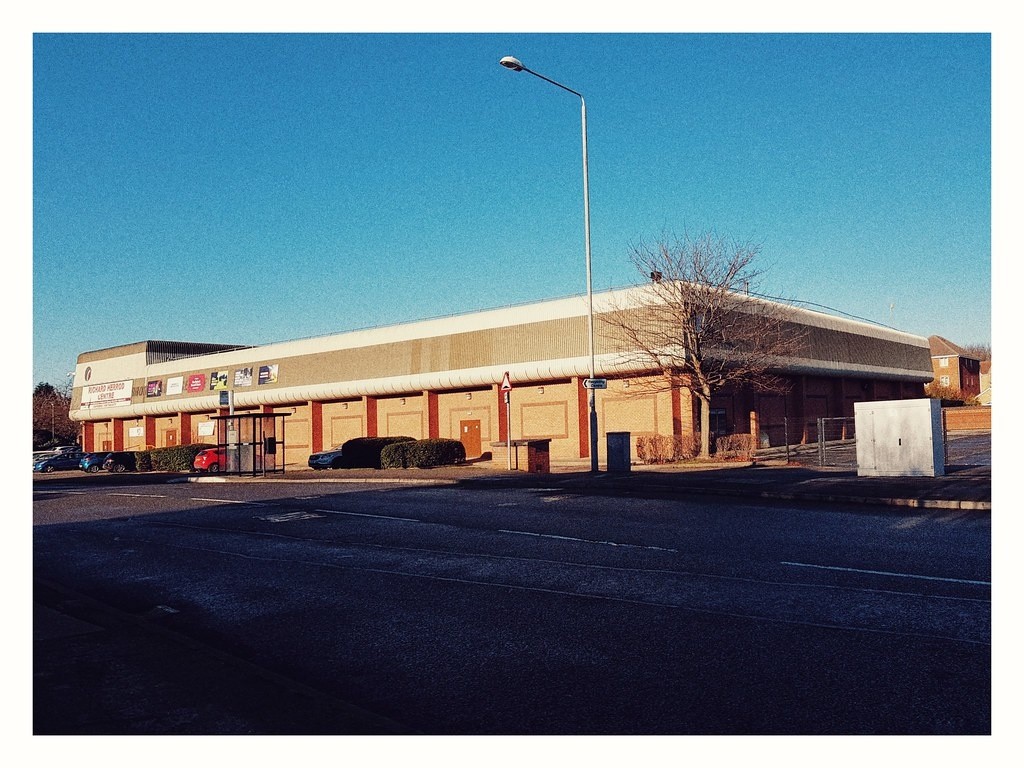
[581,378,608,389]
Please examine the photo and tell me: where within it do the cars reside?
[79,452,111,474]
[193,448,274,473]
[33,446,87,472]
[102,451,137,473]
[308,442,343,470]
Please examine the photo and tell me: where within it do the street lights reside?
[500,55,598,474]
[49,402,55,446]
